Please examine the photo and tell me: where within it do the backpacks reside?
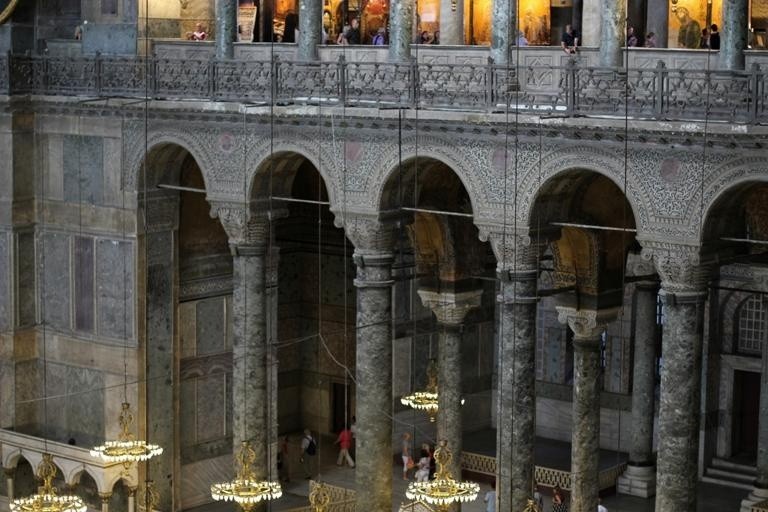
[305,436,316,456]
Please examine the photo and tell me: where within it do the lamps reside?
[405,440,480,512]
[90,403,164,462]
[400,360,465,423]
[9,453,87,512]
[210,441,283,512]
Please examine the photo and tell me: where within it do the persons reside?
[627,26,656,47]
[483,479,495,511]
[401,431,436,482]
[415,30,440,46]
[534,480,567,512]
[598,496,609,512]
[190,23,206,41]
[337,18,361,45]
[676,7,701,49]
[371,27,386,45]
[561,24,580,55]
[279,415,357,482]
[516,31,528,46]
[699,24,721,50]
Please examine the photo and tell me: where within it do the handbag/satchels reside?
[406,458,414,469]
